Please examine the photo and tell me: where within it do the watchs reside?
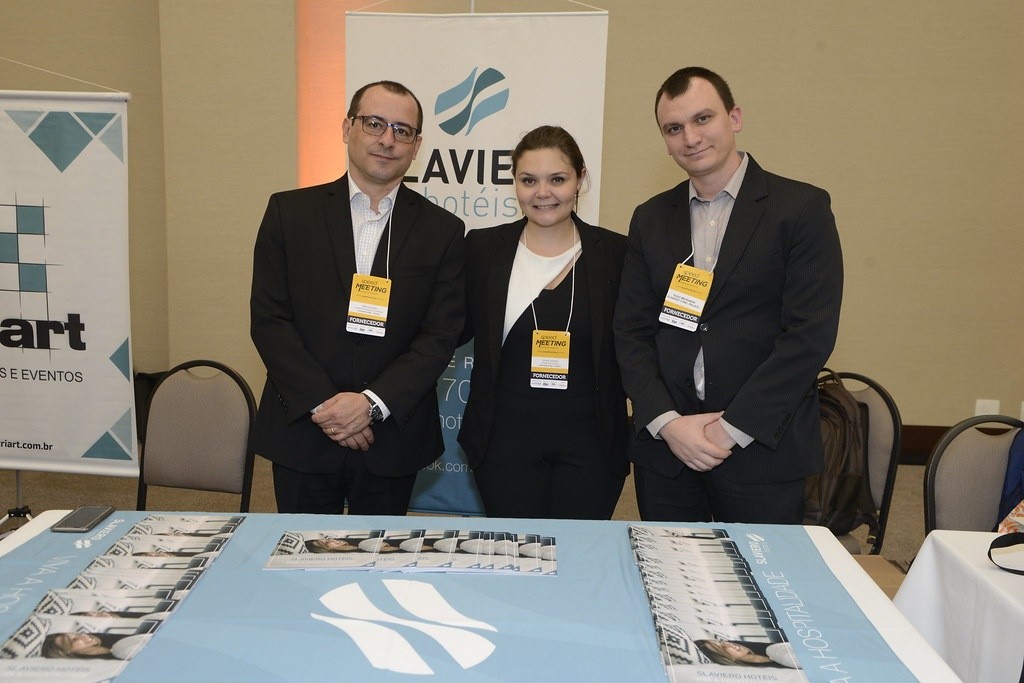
[363,394,384,423]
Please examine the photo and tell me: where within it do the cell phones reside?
[50,505,114,532]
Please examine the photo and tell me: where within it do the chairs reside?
[923,414,1024,536]
[136,358,258,514]
[819,371,904,554]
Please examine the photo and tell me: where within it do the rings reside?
[331,428,335,434]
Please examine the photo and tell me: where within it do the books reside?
[261,529,560,581]
[1,515,245,682]
[628,527,805,683]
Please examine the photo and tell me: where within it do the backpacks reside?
[800,366,881,545]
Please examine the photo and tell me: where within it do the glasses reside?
[350,116,419,144]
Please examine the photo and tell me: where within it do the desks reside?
[0,510,965,683]
[894,530,1024,683]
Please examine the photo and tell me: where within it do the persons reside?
[694,639,800,671]
[454,126,631,520]
[612,66,845,523]
[250,80,464,517]
[306,536,377,552]
[41,631,148,659]
